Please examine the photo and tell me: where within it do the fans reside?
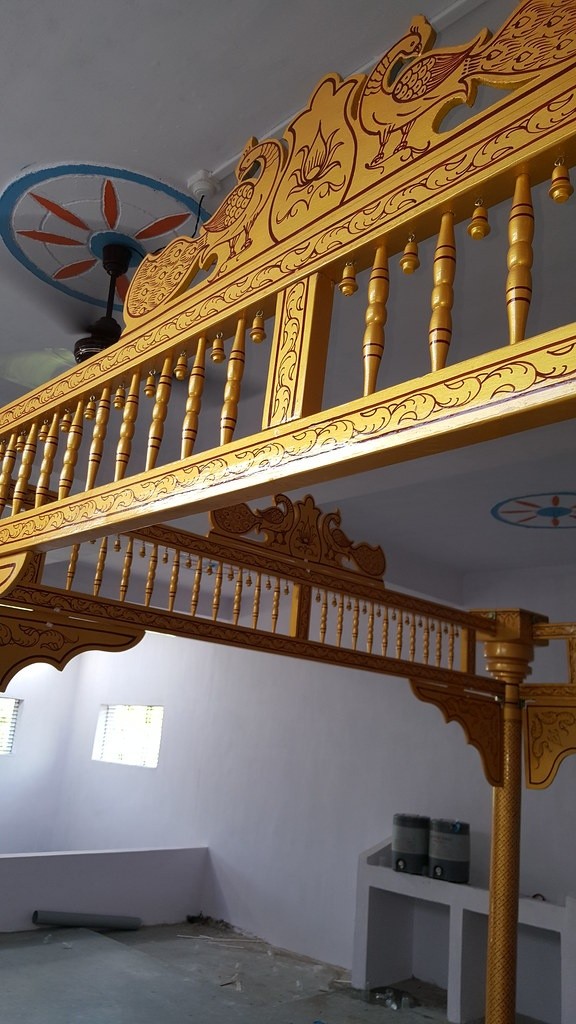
[0,244,266,407]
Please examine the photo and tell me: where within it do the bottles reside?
[391,812,430,876]
[426,818,471,885]
